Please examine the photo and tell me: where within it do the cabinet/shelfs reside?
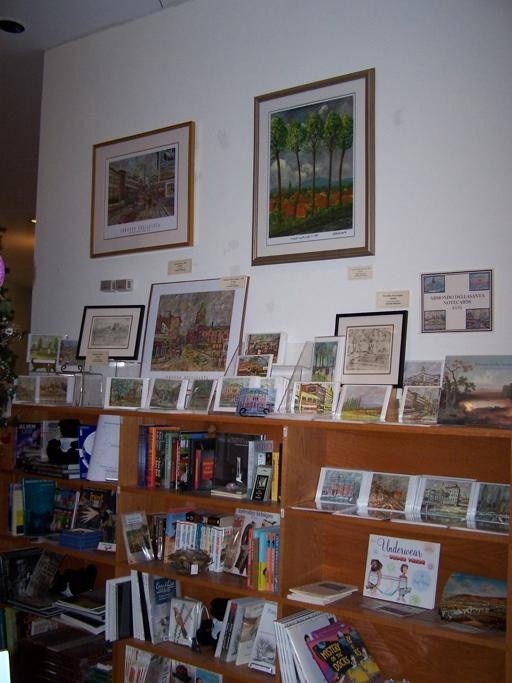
[286,403,512,682]
[117,396,284,681]
[0,401,120,681]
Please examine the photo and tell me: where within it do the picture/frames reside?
[416,266,494,334]
[25,332,61,363]
[54,338,81,371]
[74,303,146,360]
[332,308,408,388]
[88,119,195,258]
[250,66,376,266]
[138,275,250,377]
